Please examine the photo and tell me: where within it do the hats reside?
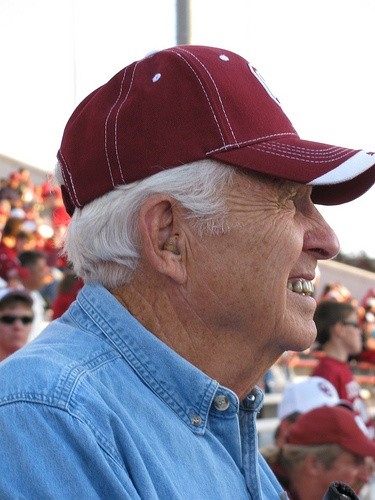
[286,406,375,457]
[56,46,375,218]
[278,375,352,419]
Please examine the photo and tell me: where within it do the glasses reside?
[2,316,32,325]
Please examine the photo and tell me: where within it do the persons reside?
[0,44,375,500]
[0,169,83,361]
[255,283,375,500]
